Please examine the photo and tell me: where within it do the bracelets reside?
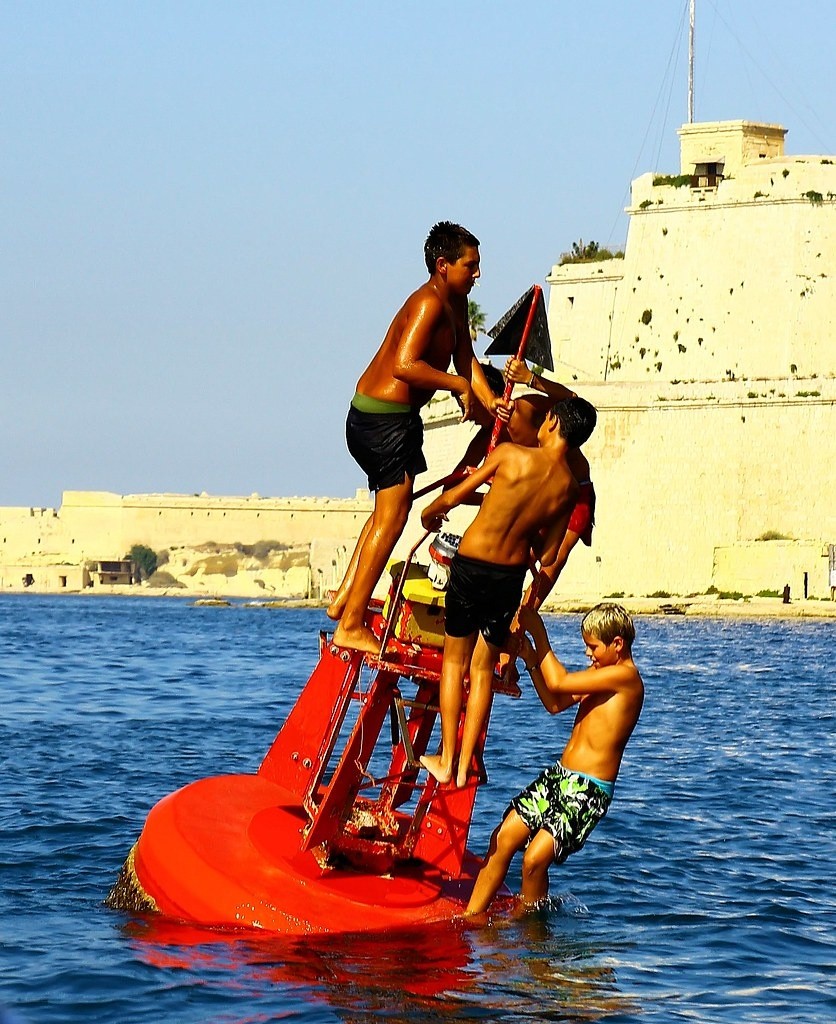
[527,372,535,387]
[523,664,538,672]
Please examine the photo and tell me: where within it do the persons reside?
[420,358,596,788]
[325,220,515,655]
[466,603,645,915]
